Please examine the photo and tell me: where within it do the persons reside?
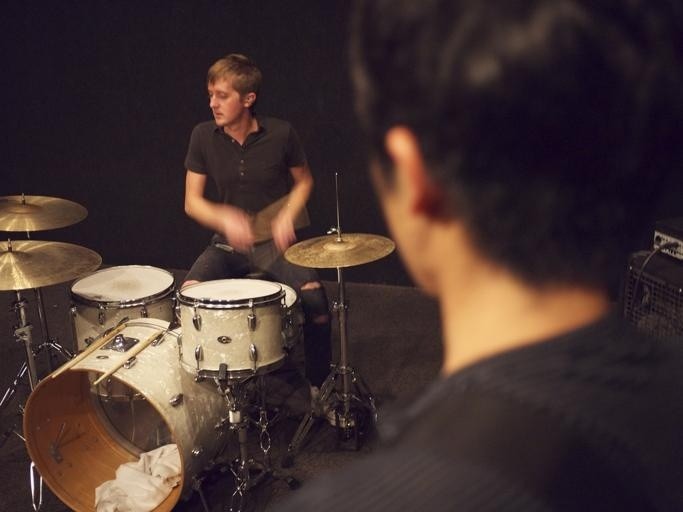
[263,0,683,512]
[175,54,357,431]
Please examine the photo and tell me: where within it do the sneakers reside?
[308,382,356,429]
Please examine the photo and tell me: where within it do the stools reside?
[237,262,306,398]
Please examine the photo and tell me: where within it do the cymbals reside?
[284,234,395,268]
[0,195,88,231]
[0,240,102,291]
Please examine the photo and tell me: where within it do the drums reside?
[70,265,175,355]
[178,279,287,379]
[278,282,306,369]
[22,317,232,512]
